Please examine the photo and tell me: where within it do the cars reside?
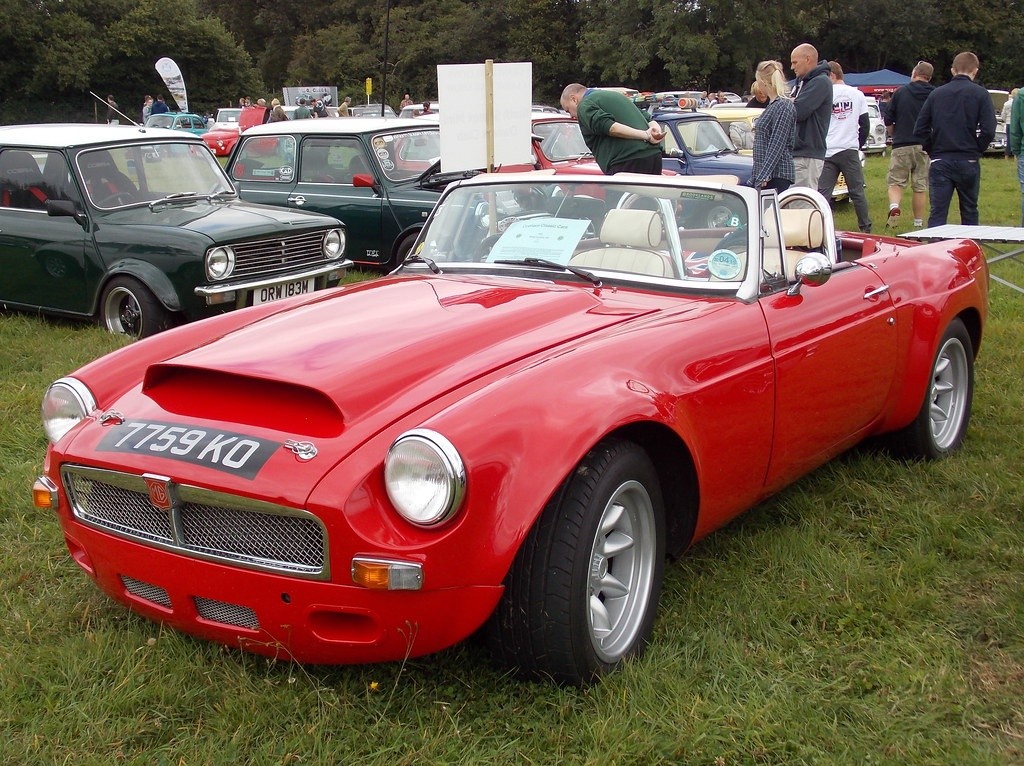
[0,124,354,340]
[145,87,885,272]
[976,90,1011,153]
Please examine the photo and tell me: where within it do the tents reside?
[785,67,911,94]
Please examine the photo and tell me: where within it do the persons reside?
[420,101,435,115]
[789,43,833,209]
[879,93,891,118]
[698,91,732,109]
[337,96,351,116]
[311,98,332,118]
[1010,86,1024,228]
[818,61,871,233]
[879,61,937,233]
[270,98,286,122]
[293,99,310,121]
[1001,88,1021,161]
[400,94,414,110]
[137,95,170,124]
[913,51,997,244]
[745,57,797,209]
[560,83,667,176]
[239,96,273,124]
[106,95,120,124]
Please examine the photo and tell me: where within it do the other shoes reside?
[886,207,901,228]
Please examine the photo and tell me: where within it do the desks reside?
[894,224,1024,294]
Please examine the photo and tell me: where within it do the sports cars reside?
[33,170,990,687]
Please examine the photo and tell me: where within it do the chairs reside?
[708,208,823,282]
[0,150,49,211]
[62,150,138,208]
[569,208,675,278]
[350,155,368,176]
